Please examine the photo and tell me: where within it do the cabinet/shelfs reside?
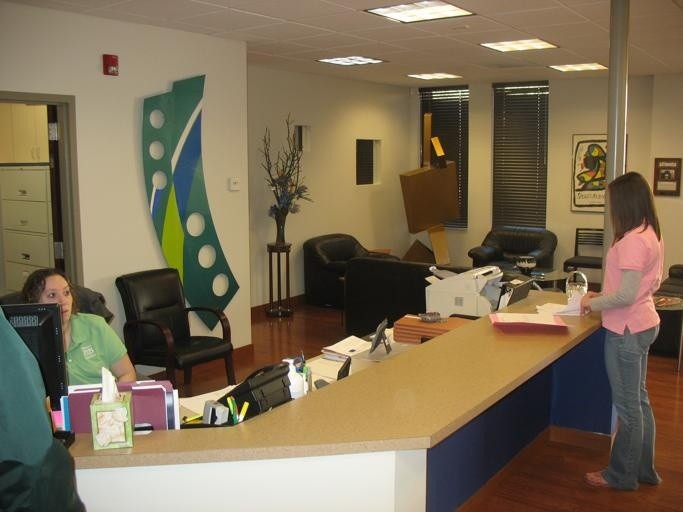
[0,101,55,295]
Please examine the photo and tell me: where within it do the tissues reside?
[89,367,133,451]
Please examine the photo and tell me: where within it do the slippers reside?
[638,476,663,486]
[585,470,638,491]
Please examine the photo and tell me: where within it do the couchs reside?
[344,257,563,338]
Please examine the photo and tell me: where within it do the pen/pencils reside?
[227,395,249,425]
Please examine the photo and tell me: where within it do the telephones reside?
[211,362,292,425]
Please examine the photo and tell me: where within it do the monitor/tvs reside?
[0,302,68,411]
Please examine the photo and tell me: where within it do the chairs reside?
[114,267,236,391]
[302,233,400,309]
[466,226,604,284]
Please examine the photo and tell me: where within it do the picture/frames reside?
[570,133,628,215]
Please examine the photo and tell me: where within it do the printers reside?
[425,265,534,318]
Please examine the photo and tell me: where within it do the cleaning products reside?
[282,356,308,398]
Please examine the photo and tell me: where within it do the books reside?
[320,334,372,363]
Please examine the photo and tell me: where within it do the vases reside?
[273,214,286,245]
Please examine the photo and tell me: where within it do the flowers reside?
[257,113,313,216]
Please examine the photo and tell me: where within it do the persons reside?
[23,268,138,387]
[579,170,665,489]
